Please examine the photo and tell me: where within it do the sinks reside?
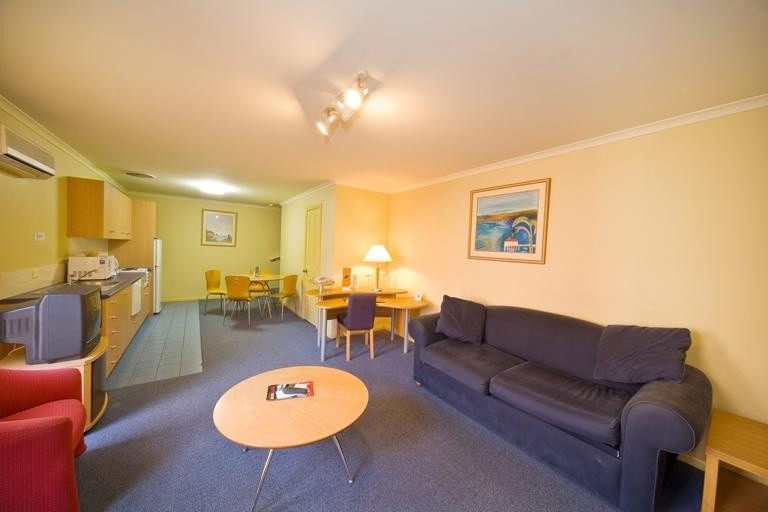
[78,281,119,291]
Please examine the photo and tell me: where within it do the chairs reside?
[336,292,377,361]
[203,267,299,327]
[1,364,88,511]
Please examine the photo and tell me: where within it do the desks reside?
[306,286,428,362]
[214,363,370,512]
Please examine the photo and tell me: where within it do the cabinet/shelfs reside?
[100,271,151,379]
[66,175,132,240]
[1,337,108,434]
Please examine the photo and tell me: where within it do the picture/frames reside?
[201,207,238,248]
[466,176,554,265]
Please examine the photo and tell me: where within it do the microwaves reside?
[68,255,115,279]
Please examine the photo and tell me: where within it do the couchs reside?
[409,304,712,512]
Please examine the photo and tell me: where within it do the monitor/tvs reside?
[0,285,102,364]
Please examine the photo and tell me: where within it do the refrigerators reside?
[154,239,163,314]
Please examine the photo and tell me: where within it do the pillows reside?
[592,323,693,385]
[434,293,487,347]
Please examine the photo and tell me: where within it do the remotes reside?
[282,387,308,394]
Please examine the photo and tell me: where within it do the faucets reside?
[69,270,97,285]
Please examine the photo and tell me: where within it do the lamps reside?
[363,245,392,292]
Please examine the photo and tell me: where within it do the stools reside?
[701,409,768,512]
